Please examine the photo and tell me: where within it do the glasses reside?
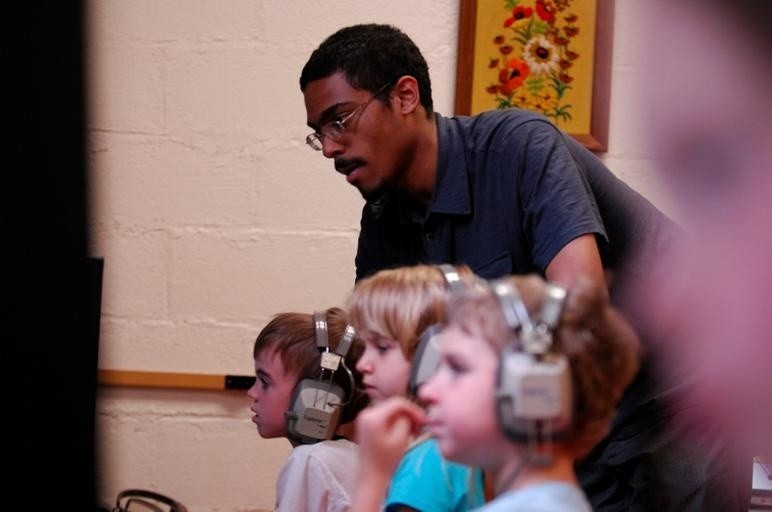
[305,82,390,151]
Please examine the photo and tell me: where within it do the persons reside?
[247,307,367,512]
[418,274,641,511]
[295,23,756,510]
[346,264,488,510]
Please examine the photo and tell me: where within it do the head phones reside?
[409,263,462,398]
[285,311,356,445]
[489,279,575,442]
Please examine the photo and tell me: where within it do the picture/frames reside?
[454,1,610,156]
